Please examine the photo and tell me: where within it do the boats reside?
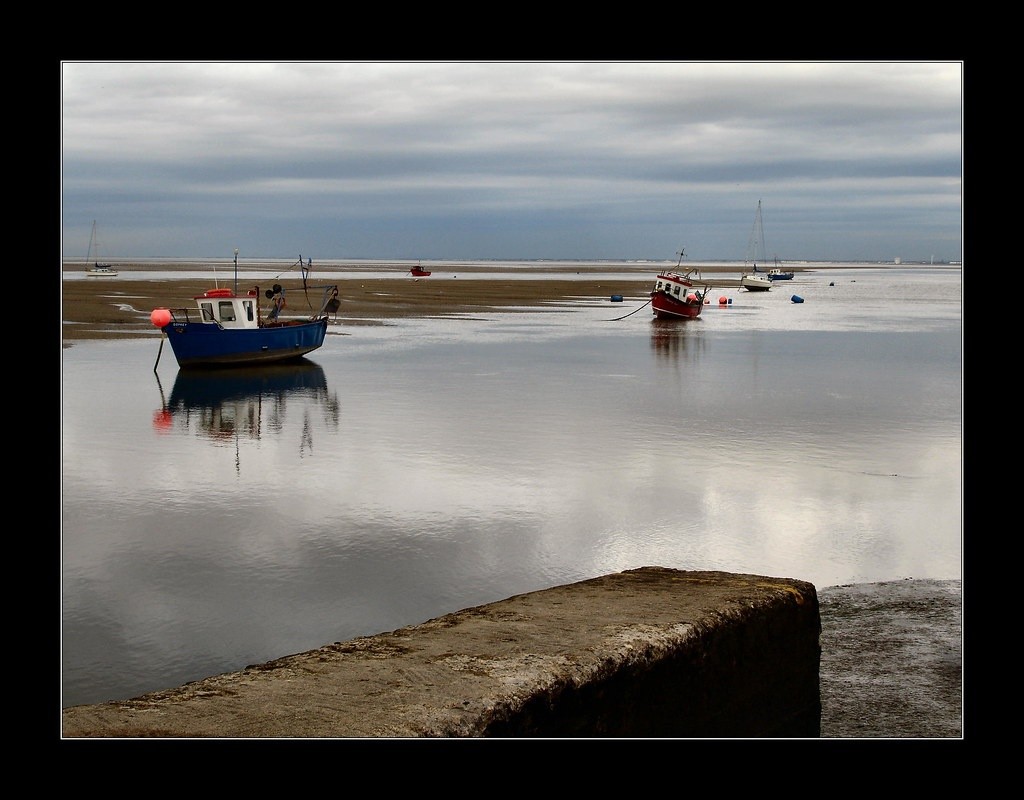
[411,256,431,276]
[151,248,342,366]
[155,358,339,474]
[650,248,712,320]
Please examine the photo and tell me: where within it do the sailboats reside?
[768,256,794,280]
[740,200,773,291]
[81,220,118,276]
